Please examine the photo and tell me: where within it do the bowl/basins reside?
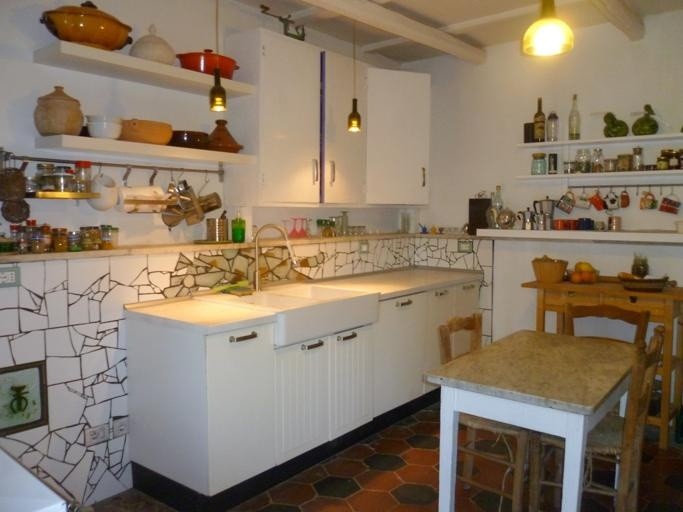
[170,130,208,150]
[566,269,601,285]
[86,113,122,139]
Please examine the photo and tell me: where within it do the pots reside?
[121,118,172,144]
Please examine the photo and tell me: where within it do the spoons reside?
[3,161,30,223]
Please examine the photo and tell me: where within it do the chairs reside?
[437,311,532,511]
[558,302,651,415]
[529,324,666,511]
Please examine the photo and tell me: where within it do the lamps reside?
[209,2,229,111]
[348,24,363,133]
[520,0,575,58]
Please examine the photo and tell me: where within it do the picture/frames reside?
[0,360,51,439]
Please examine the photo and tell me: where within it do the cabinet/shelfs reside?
[430,281,481,406]
[376,291,428,430]
[225,26,367,211]
[273,323,374,485]
[122,328,274,512]
[367,64,430,210]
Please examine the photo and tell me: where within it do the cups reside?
[299,216,307,239]
[290,217,298,239]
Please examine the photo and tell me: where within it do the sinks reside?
[194,282,382,348]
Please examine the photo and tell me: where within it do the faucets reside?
[254,223,288,294]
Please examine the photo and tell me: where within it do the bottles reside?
[578,149,590,172]
[69,170,80,192]
[26,219,36,251]
[41,225,51,248]
[606,157,615,170]
[53,165,71,190]
[76,160,92,191]
[487,192,499,229]
[68,230,78,250]
[53,227,64,251]
[532,153,549,175]
[632,145,643,170]
[657,155,668,170]
[534,97,545,141]
[37,163,53,192]
[546,110,558,139]
[609,215,621,231]
[594,148,604,172]
[81,228,99,249]
[569,94,580,139]
[495,184,505,229]
[548,153,559,173]
[101,224,112,250]
[18,227,26,254]
[108,225,118,246]
[24,176,39,193]
[618,153,630,170]
[31,228,44,253]
[9,225,16,250]
[660,149,668,157]
[563,155,576,172]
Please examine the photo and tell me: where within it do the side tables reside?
[521,275,683,447]
